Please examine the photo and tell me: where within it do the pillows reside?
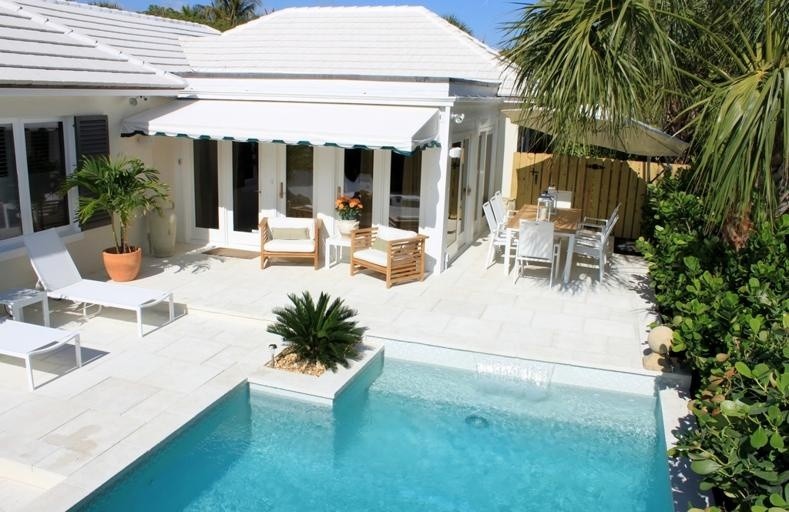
[371,238,388,252]
[271,227,307,240]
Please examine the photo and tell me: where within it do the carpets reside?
[200,247,261,259]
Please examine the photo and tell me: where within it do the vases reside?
[336,219,359,235]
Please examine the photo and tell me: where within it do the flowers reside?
[335,195,364,220]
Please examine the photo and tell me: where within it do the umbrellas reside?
[498,103,692,162]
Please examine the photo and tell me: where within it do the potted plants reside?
[54,153,174,282]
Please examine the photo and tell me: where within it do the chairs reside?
[24,228,174,338]
[350,225,430,289]
[257,217,323,271]
[289,204,312,218]
[0,316,82,391]
[389,215,419,230]
[481,190,623,289]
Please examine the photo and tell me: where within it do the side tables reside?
[0,287,50,327]
[325,235,351,271]
[0,200,16,229]
